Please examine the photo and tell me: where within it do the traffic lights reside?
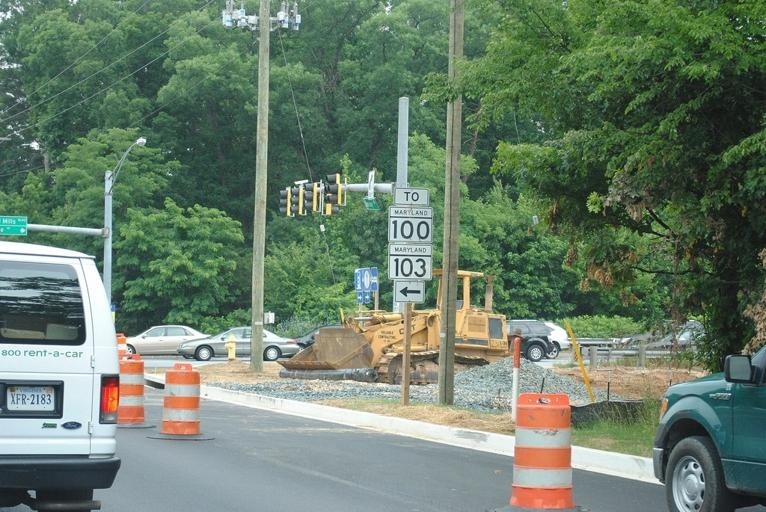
[275,171,349,218]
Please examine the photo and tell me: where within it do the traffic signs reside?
[387,244,434,279]
[388,205,434,245]
[0,214,27,236]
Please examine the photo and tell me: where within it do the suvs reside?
[648,347,765,512]
[617,318,706,354]
[543,323,571,361]
[293,322,350,354]
[502,319,556,362]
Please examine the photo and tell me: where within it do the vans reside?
[0,240,120,512]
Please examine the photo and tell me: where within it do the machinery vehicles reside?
[277,265,510,389]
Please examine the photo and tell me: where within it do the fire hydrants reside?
[222,334,237,363]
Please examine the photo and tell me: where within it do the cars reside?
[124,324,212,360]
[177,326,303,363]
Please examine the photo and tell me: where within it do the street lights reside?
[103,136,151,313]
